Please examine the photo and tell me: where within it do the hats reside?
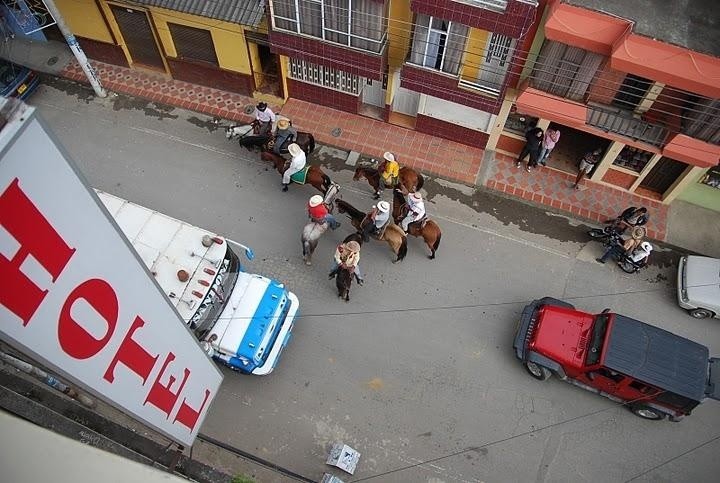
[384,152,394,162]
[257,102,268,112]
[346,241,361,252]
[377,201,390,213]
[408,192,422,203]
[632,227,644,240]
[309,195,323,208]
[641,241,653,254]
[277,119,289,129]
[287,143,300,157]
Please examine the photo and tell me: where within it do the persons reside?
[272,119,297,153]
[395,186,426,236]
[254,100,277,138]
[514,125,542,173]
[372,152,400,196]
[615,240,654,266]
[306,194,343,231]
[569,145,602,190]
[327,241,365,287]
[280,143,307,192]
[596,218,648,265]
[359,200,391,243]
[538,124,561,168]
[603,203,652,234]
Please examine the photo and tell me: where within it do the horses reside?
[391,182,442,261]
[334,197,409,264]
[299,181,343,267]
[237,131,316,159]
[224,114,294,135]
[353,165,425,198]
[258,146,331,198]
[328,230,366,302]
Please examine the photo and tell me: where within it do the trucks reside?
[89,186,302,382]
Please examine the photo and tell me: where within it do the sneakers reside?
[596,258,606,264]
[327,275,335,280]
[377,190,383,195]
[357,278,365,286]
[332,222,340,230]
[516,161,531,173]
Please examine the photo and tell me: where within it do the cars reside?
[0,56,42,103]
[675,252,720,322]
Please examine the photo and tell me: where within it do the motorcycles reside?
[586,215,653,276]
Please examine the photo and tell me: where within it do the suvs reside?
[509,292,720,425]
